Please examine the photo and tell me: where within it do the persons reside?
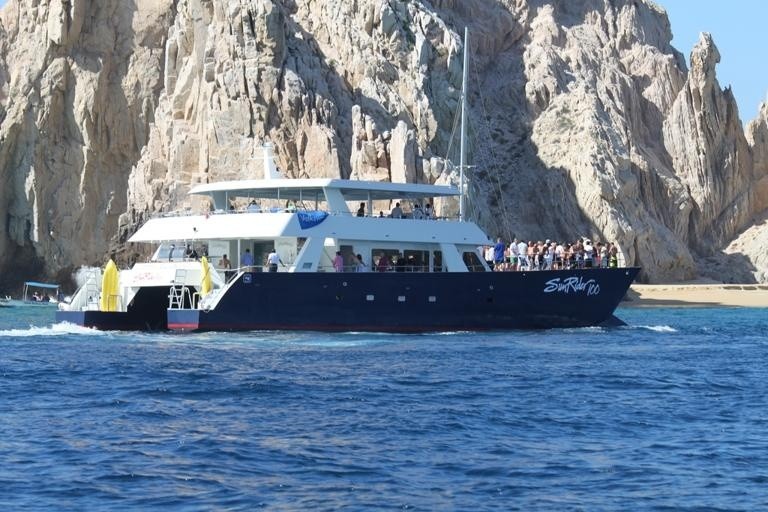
[391,203,402,219]
[378,212,385,218]
[241,248,254,272]
[247,200,262,213]
[229,206,237,215]
[333,251,344,273]
[265,248,286,272]
[348,236,617,272]
[414,204,424,219]
[425,204,433,219]
[357,203,365,216]
[169,243,208,261]
[219,254,231,283]
[32,288,64,302]
[286,200,297,213]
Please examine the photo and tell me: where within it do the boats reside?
[55,26,642,335]
[0,282,64,308]
